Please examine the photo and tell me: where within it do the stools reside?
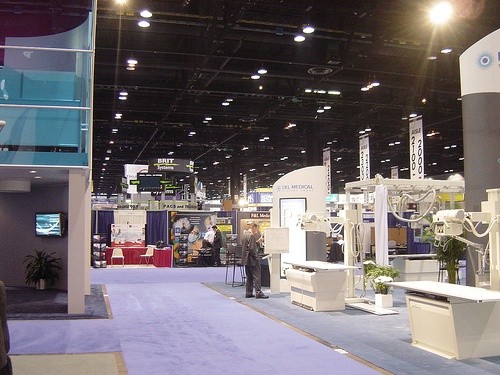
[225,245,246,288]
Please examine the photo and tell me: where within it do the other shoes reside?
[256,295,269,299]
[246,295,255,298]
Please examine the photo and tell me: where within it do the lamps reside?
[0,178,31,193]
[293,10,314,42]
[360,73,380,91]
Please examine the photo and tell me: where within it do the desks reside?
[384,281,500,360]
[106,246,171,267]
[283,261,358,311]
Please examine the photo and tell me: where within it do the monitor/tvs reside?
[34,211,64,237]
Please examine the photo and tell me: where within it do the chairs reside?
[111,249,125,267]
[138,248,154,267]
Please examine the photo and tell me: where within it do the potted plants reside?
[365,265,399,308]
[23,249,62,290]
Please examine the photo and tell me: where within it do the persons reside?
[211,225,222,267]
[336,234,344,245]
[240,223,269,299]
